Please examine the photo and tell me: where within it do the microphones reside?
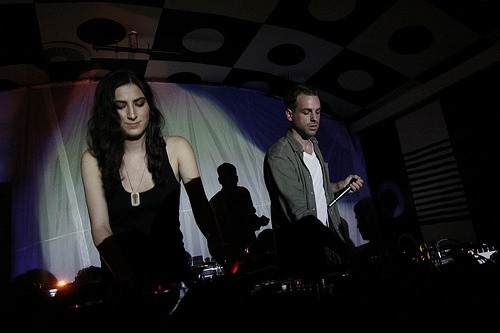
[327,177,357,207]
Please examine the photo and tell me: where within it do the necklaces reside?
[122,158,149,207]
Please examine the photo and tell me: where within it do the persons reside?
[79,67,223,293]
[265,83,364,273]
